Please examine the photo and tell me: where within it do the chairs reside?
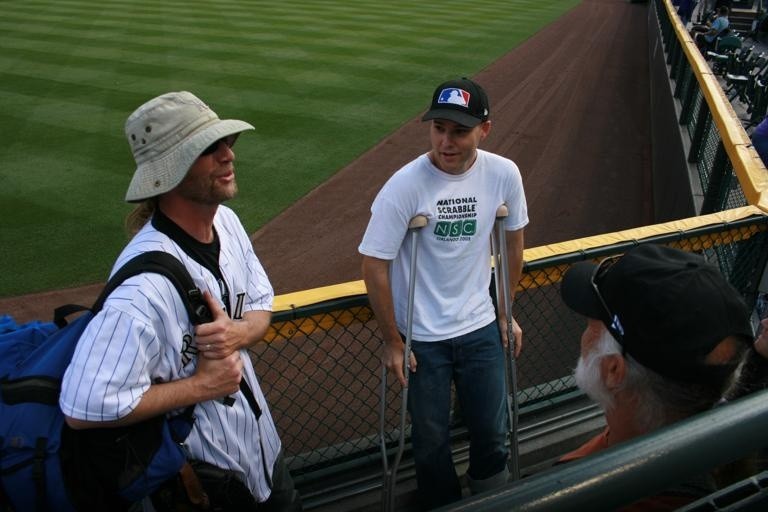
[707,29,768,130]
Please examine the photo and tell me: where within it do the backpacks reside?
[1,248,214,511]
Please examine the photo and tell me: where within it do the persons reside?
[750,113,768,163]
[356,75,531,505]
[559,240,751,466]
[60,88,305,512]
[753,314,768,358]
[695,5,730,50]
[675,0,698,28]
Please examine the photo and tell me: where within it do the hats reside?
[420,77,491,130]
[559,240,758,404]
[124,91,256,204]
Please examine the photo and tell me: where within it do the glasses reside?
[199,132,241,157]
[587,251,629,334]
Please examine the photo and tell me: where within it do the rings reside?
[205,343,214,352]
